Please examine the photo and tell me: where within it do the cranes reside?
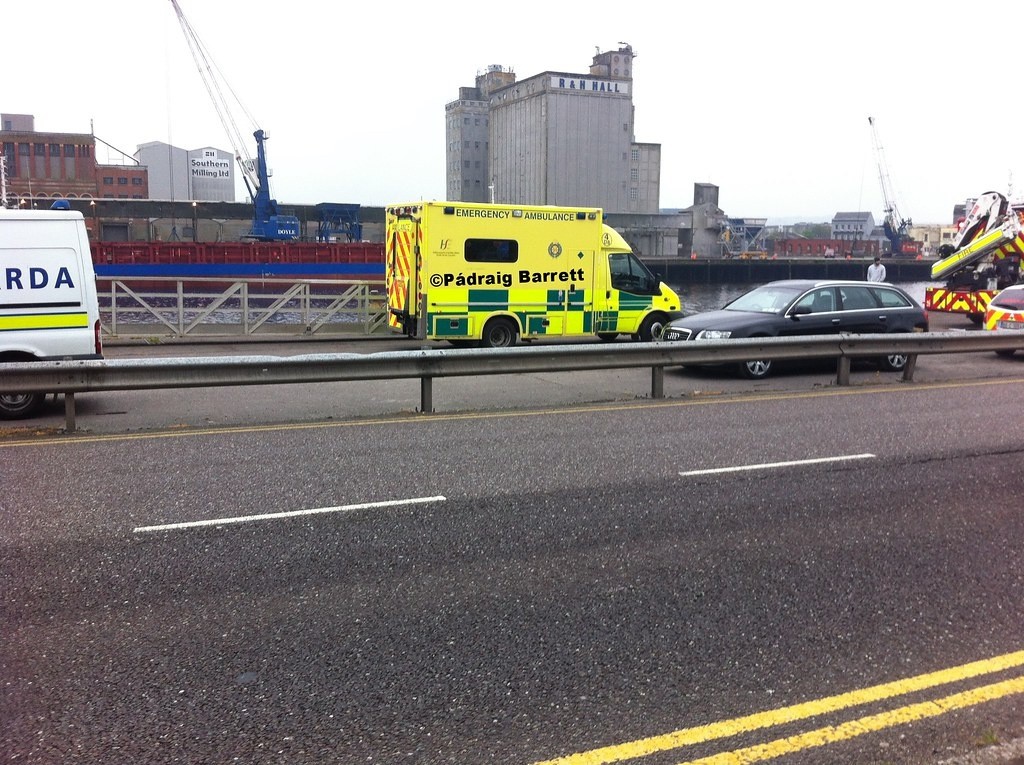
[869,116,912,254]
[170,0,301,241]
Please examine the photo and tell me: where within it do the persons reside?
[691,251,696,259]
[917,253,922,260]
[867,257,886,304]
[772,253,777,259]
[845,248,853,258]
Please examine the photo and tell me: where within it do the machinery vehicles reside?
[924,191,1024,327]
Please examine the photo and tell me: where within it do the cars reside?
[982,284,1024,354]
[658,278,930,380]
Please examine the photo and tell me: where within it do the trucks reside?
[384,202,681,349]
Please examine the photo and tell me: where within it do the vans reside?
[0,200,104,420]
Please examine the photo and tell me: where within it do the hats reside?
[874,257,880,262]
